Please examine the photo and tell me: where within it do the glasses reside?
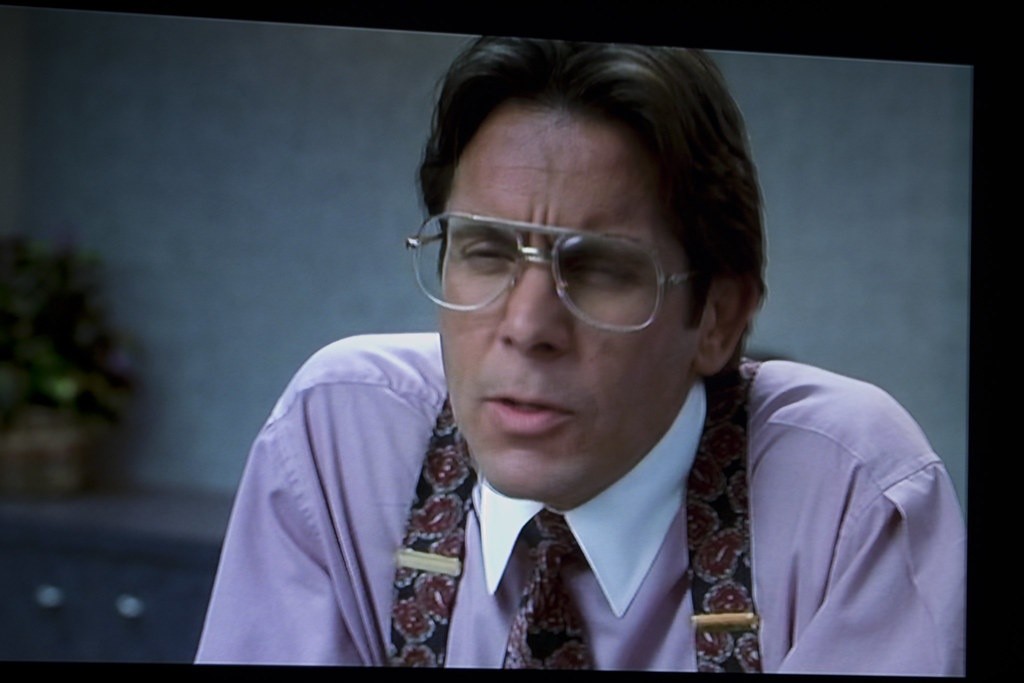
[406,210,697,335]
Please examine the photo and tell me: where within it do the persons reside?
[194,31,967,678]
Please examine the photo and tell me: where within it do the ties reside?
[499,510,594,671]
